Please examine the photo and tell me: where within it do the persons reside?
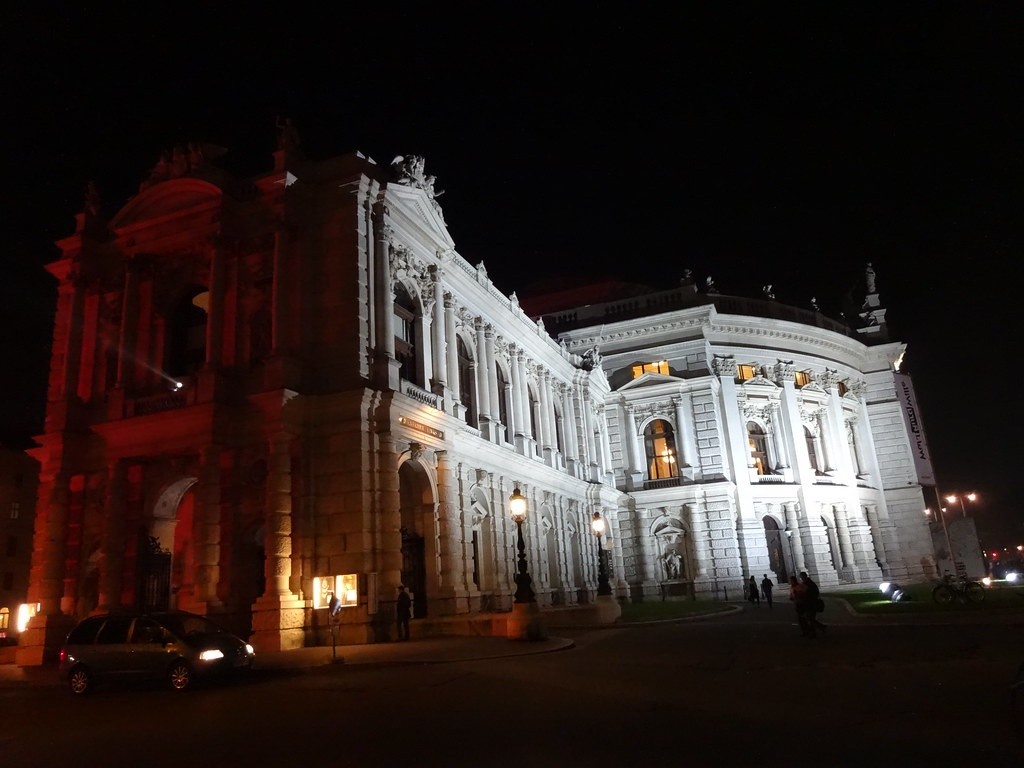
[396,586,411,641]
[761,574,773,608]
[749,576,760,609]
[789,570,828,639]
[665,550,682,579]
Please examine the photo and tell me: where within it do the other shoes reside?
[807,634,816,640]
[821,625,827,632]
[799,633,808,638]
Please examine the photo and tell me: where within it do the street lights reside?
[592,510,621,623]
[506,484,549,640]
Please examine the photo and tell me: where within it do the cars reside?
[58,610,258,697]
[998,557,1024,581]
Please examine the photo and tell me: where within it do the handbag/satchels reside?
[814,598,825,613]
[762,592,766,601]
[748,594,754,602]
